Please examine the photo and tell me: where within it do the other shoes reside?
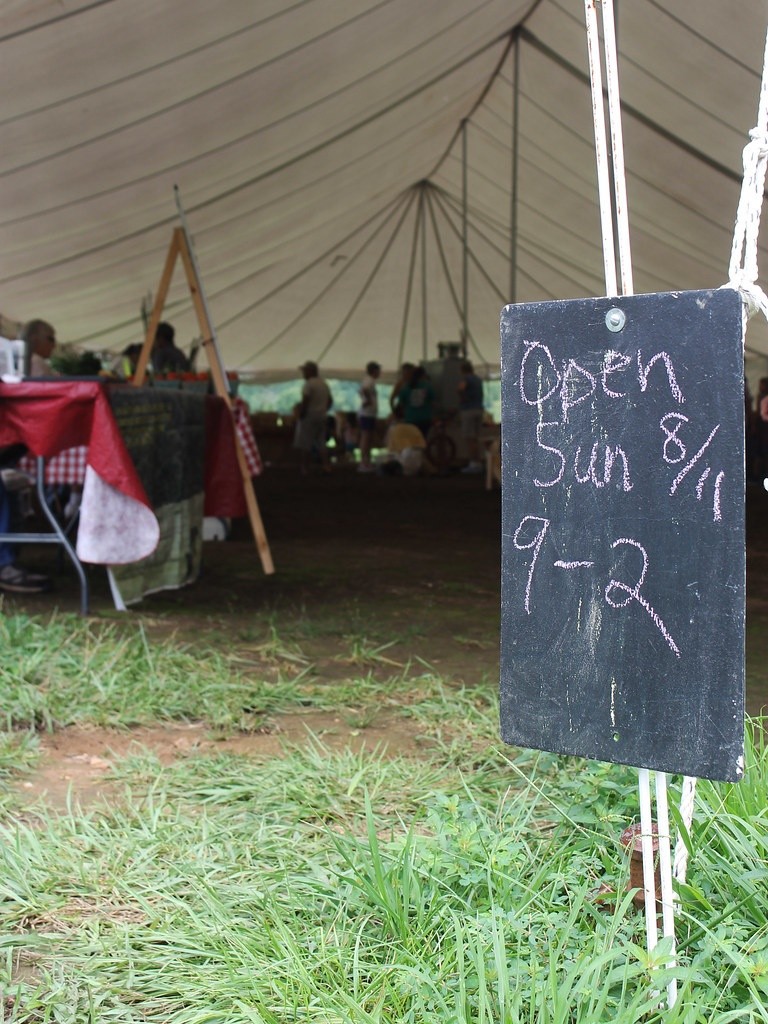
[302,469,310,476]
[322,468,331,474]
[0,569,51,594]
[360,461,377,471]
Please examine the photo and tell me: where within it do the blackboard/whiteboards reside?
[495,284,752,781]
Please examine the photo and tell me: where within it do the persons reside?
[292,343,767,497]
[111,321,192,381]
[0,318,56,595]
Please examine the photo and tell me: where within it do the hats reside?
[298,361,318,371]
